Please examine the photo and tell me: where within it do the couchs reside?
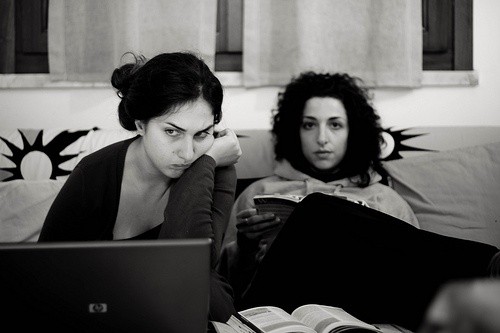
[0,125,500,248]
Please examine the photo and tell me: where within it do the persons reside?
[36,51,243,325]
[218,71,421,304]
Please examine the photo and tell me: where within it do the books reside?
[252,193,349,242]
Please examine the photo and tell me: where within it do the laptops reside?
[0,237,214,333]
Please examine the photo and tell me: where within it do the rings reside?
[245,219,248,224]
